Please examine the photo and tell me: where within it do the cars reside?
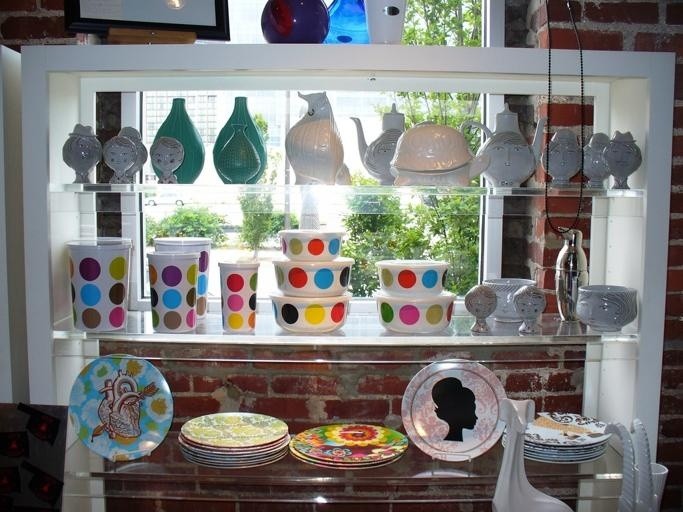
[144,191,193,206]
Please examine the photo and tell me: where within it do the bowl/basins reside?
[481,276,540,326]
[576,283,638,331]
[271,220,457,336]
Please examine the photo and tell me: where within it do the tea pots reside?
[352,102,410,188]
[458,100,548,189]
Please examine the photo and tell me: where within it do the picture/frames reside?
[65,0,231,42]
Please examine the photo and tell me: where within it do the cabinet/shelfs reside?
[20,42,677,511]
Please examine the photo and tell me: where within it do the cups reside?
[633,461,669,512]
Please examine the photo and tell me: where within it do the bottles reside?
[553,225,589,324]
[144,235,213,334]
[218,259,260,336]
[150,97,204,185]
[211,96,268,184]
[64,234,133,334]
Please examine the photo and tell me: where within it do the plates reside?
[66,351,175,463]
[499,409,617,466]
[176,407,411,471]
[399,357,509,465]
